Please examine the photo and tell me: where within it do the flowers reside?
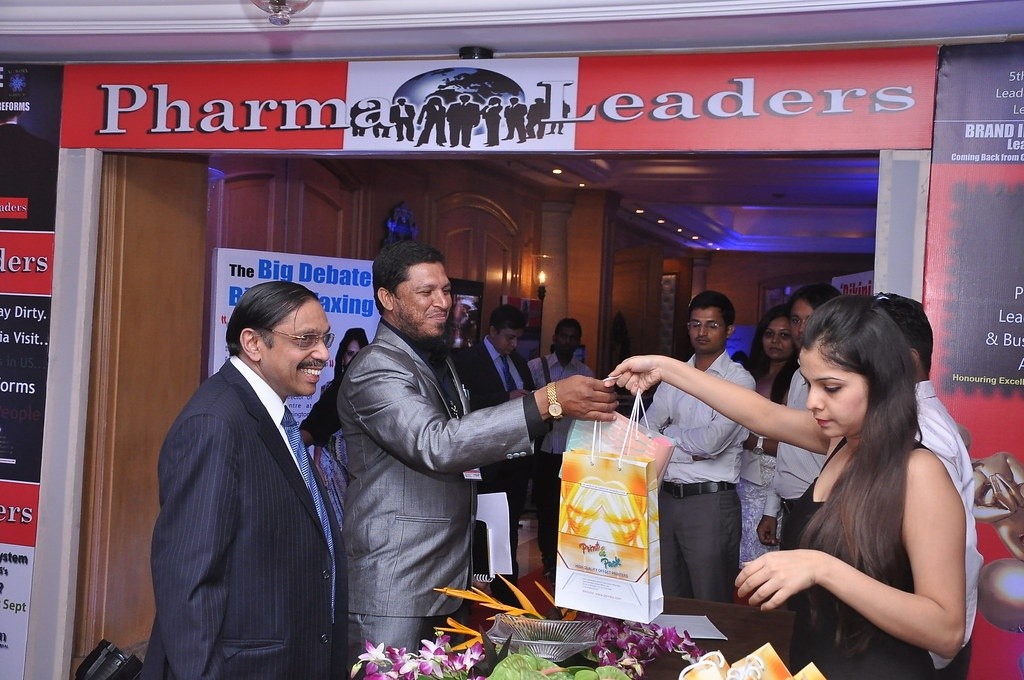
[351,570,705,680]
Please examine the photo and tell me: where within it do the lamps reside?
[532,247,556,300]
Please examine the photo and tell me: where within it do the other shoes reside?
[542,558,554,577]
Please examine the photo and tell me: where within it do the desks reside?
[630,598,797,680]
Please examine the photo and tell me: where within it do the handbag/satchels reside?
[558,374,676,495]
[553,373,664,624]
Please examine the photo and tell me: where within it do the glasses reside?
[687,321,726,331]
[261,326,335,348]
[872,291,891,312]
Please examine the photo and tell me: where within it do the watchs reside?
[547,382,564,419]
[753,437,764,454]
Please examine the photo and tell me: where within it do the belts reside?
[662,479,740,498]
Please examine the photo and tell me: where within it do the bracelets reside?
[659,424,669,434]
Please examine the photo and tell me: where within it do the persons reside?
[873,292,984,680]
[337,239,620,679]
[140,280,349,680]
[756,283,842,549]
[639,290,756,604]
[604,294,966,680]
[319,328,370,530]
[529,318,596,564]
[454,305,553,596]
[735,302,798,568]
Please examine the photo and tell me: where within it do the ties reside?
[500,353,518,391]
[280,405,335,625]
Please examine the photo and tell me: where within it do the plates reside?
[485,613,602,662]
